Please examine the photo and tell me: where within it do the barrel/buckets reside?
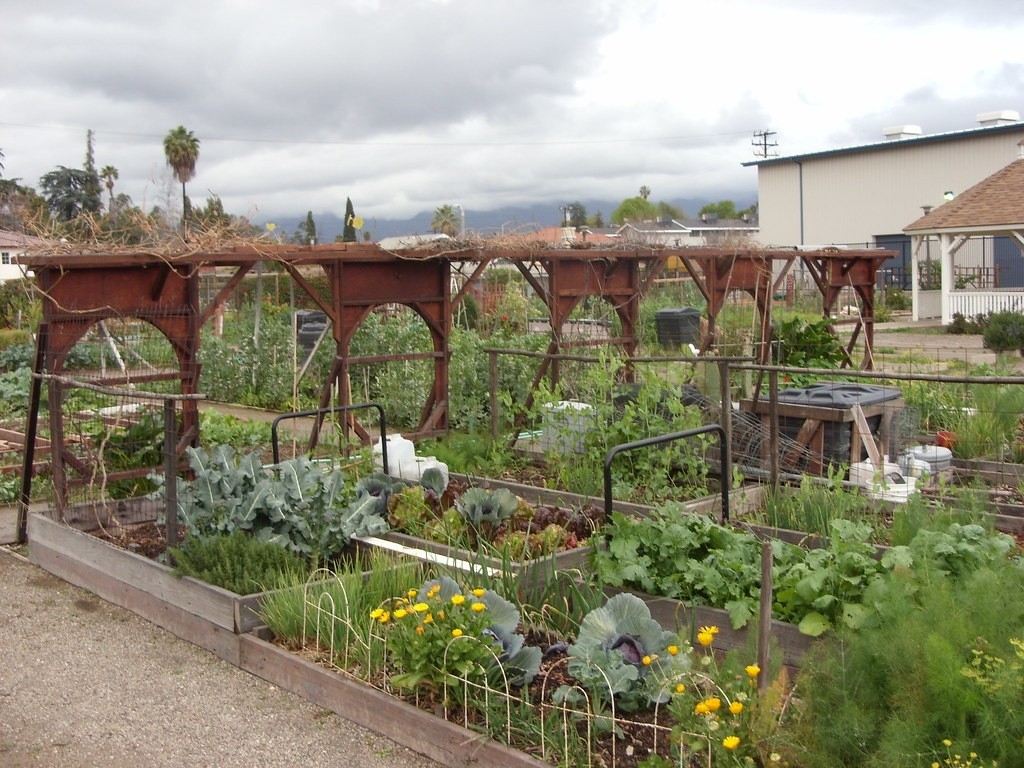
[371,434,416,486]
[407,455,450,491]
[849,430,958,504]
